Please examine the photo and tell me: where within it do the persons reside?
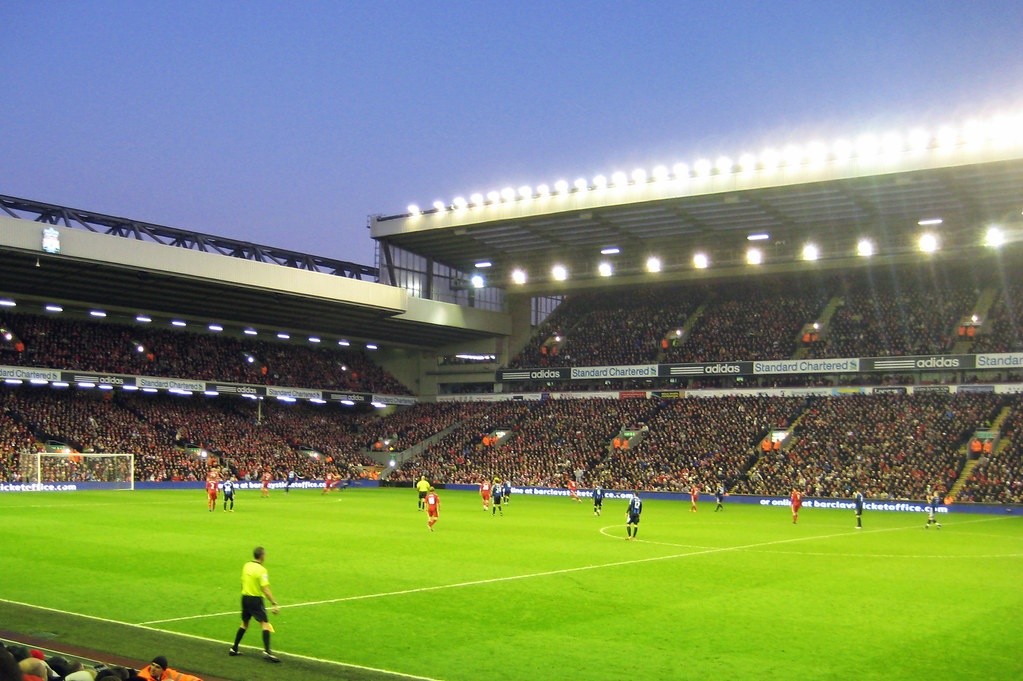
[0,256,1023,513]
[922,498,942,530]
[490,477,503,517]
[424,487,440,532]
[592,482,605,517]
[853,489,863,529]
[791,485,802,524]
[229,547,281,663]
[0,640,204,681]
[625,491,642,539]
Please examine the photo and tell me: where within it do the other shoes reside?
[263,650,280,662]
[229,647,244,656]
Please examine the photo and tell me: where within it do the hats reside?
[152,656,168,671]
